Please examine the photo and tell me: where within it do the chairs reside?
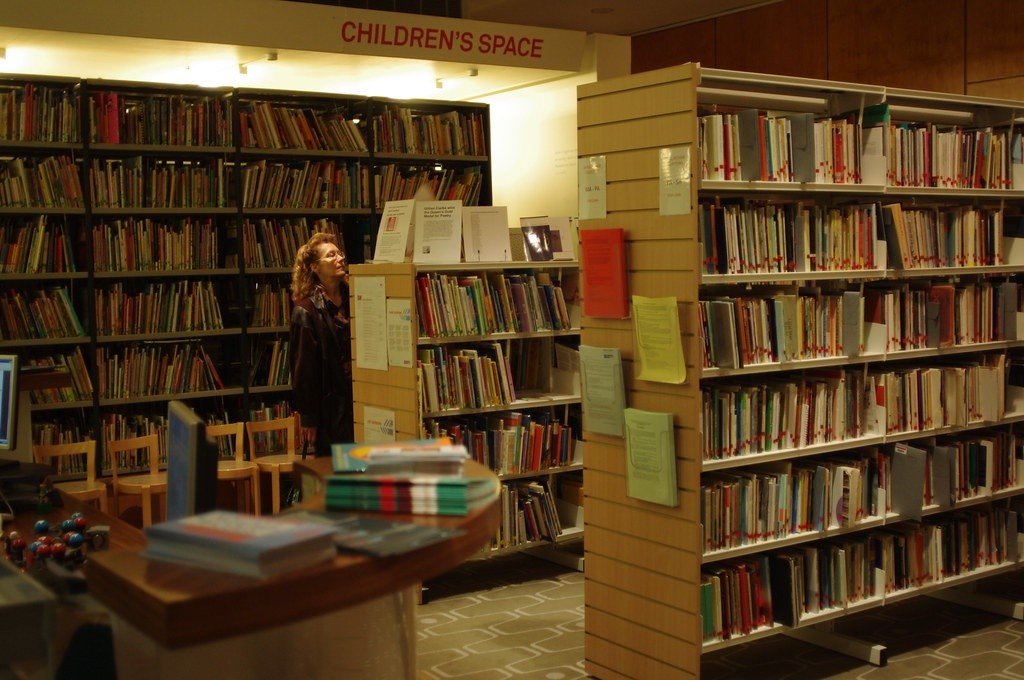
[107,435,167,529]
[246,417,315,517]
[33,440,107,514]
[205,421,261,517]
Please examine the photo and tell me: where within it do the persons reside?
[285,231,353,456]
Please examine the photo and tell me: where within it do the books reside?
[698,113,1024,641]
[143,509,470,577]
[415,273,572,550]
[0,83,486,475]
[324,438,496,516]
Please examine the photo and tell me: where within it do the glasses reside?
[317,249,347,263]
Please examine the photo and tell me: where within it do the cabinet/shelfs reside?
[0,72,493,482]
[576,61,1024,680]
[349,263,585,606]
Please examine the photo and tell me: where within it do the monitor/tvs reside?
[0,355,20,468]
[164,401,218,521]
